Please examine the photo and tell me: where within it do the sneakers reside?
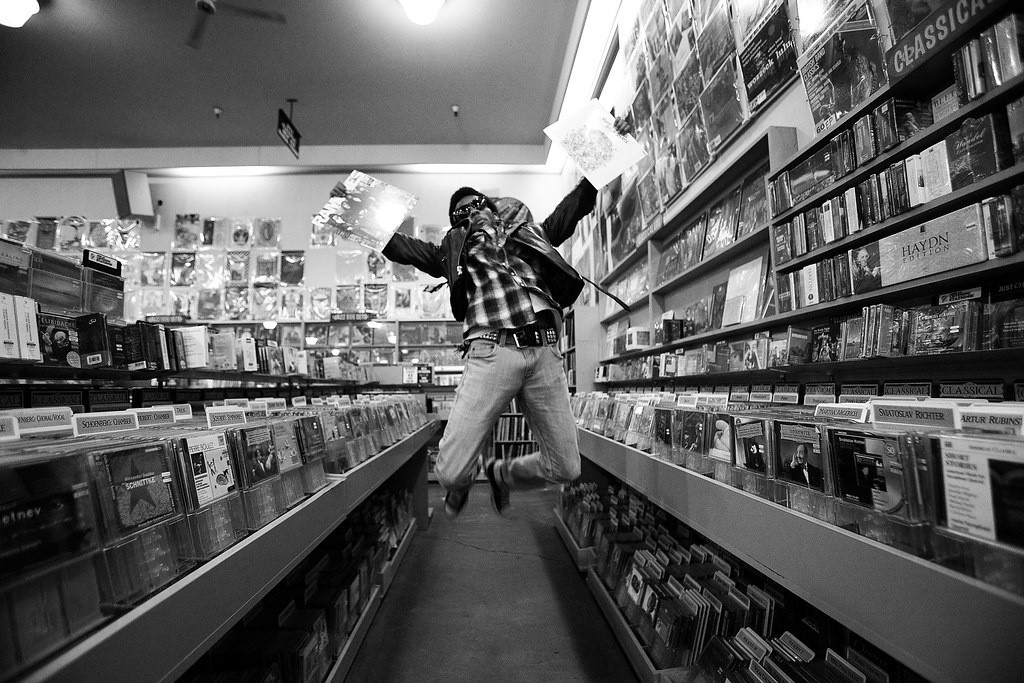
[444,462,481,517]
[484,459,511,519]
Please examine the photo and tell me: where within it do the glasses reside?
[452,195,488,223]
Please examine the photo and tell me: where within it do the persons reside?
[641,593,655,621]
[330,186,384,244]
[853,249,882,292]
[252,448,267,478]
[729,349,742,362]
[330,117,632,520]
[785,444,823,487]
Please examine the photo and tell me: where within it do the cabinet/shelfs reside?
[0,0,1024,683]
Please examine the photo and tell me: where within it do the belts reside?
[477,329,558,349]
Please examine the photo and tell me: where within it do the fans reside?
[181,0,287,50]
